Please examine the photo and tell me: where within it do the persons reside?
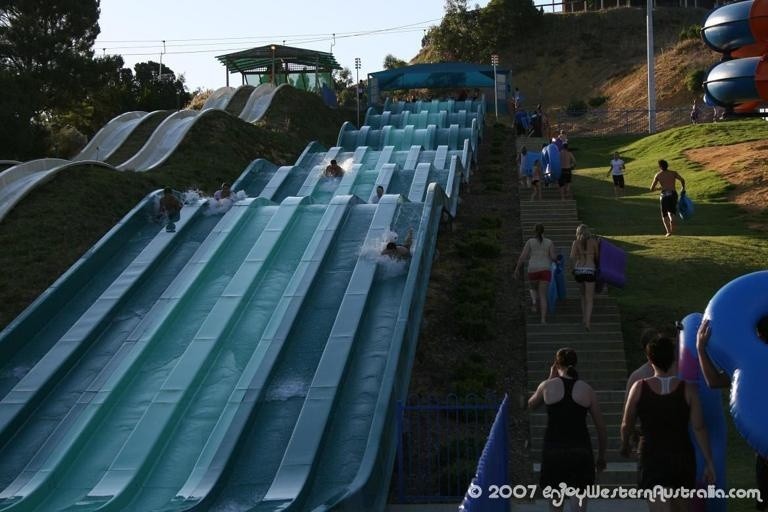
[326,159,343,177]
[381,227,414,261]
[650,159,686,239]
[569,223,600,331]
[606,150,626,198]
[511,101,578,204]
[623,324,669,463]
[371,184,385,204]
[156,186,184,233]
[358,79,365,103]
[382,88,478,104]
[214,181,235,204]
[696,315,767,512]
[526,345,608,512]
[689,99,701,127]
[711,108,718,125]
[619,333,719,511]
[510,87,520,110]
[514,222,561,324]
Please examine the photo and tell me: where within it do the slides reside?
[2,83,487,511]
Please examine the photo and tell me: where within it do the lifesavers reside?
[701,270,768,456]
[677,196,692,221]
[678,313,727,502]
[541,144,561,179]
[548,260,565,314]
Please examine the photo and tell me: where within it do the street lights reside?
[490,53,500,122]
[354,57,363,129]
